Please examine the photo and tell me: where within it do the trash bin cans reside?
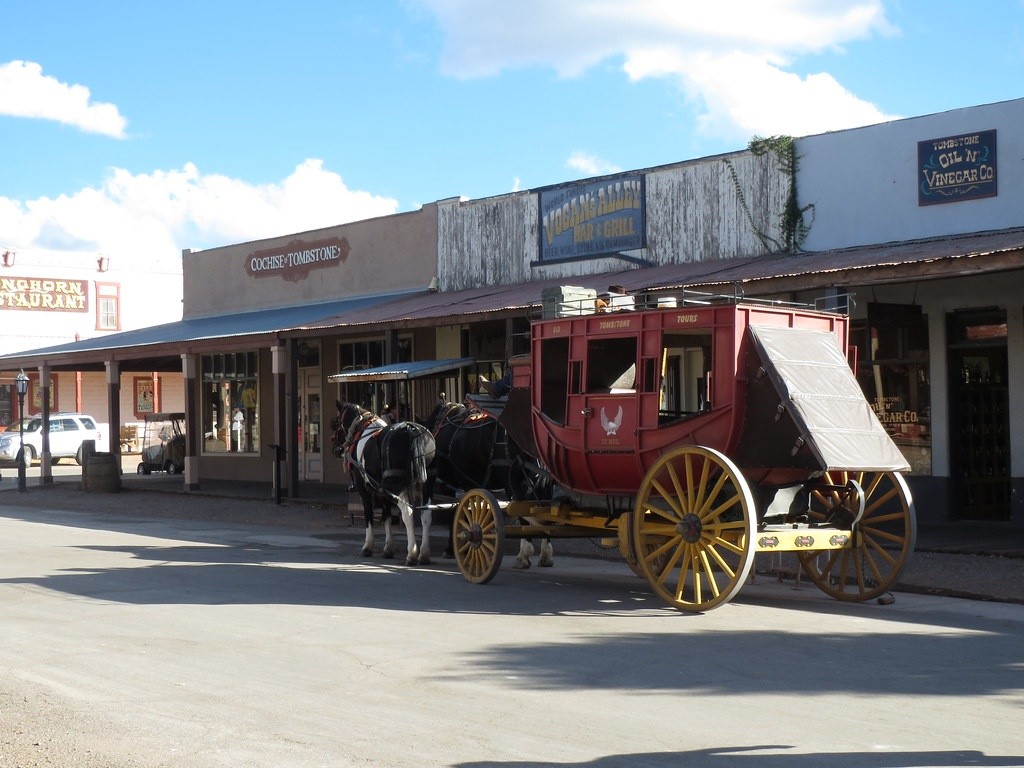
[87,451,122,492]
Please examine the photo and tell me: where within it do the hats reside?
[233,404,240,408]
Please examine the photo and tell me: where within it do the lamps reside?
[429,276,438,291]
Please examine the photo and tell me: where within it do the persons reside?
[232,404,244,449]
[479,372,512,399]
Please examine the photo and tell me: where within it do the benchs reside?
[505,333,532,389]
[120,425,138,451]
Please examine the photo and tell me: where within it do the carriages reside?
[330,293,918,614]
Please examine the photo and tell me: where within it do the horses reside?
[332,397,555,569]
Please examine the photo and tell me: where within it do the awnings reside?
[0,227,1024,370]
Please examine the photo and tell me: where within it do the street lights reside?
[15,368,30,492]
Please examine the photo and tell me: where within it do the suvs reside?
[0,411,101,469]
